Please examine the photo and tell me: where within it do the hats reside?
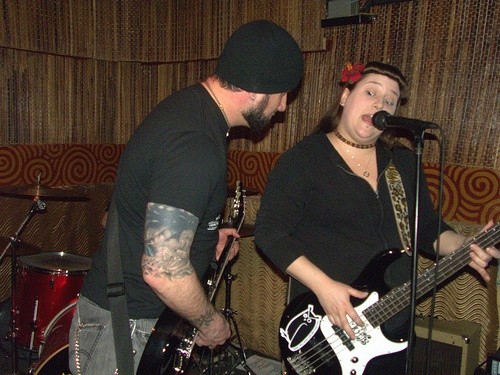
[214,19,305,94]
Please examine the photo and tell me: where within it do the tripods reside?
[198,271,257,375]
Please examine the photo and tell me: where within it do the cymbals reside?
[0,184,89,198]
[0,235,41,257]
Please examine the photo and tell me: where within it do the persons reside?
[254,62,500,375]
[68,20,303,375]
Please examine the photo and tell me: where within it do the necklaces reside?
[347,150,374,176]
[333,130,377,149]
[204,81,232,137]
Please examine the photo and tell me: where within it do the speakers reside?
[408,314,481,375]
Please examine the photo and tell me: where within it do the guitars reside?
[147,179,247,374]
[278,217,500,375]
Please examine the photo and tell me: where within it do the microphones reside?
[371,110,439,131]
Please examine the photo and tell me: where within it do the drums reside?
[29,327,74,375]
[8,249,95,353]
